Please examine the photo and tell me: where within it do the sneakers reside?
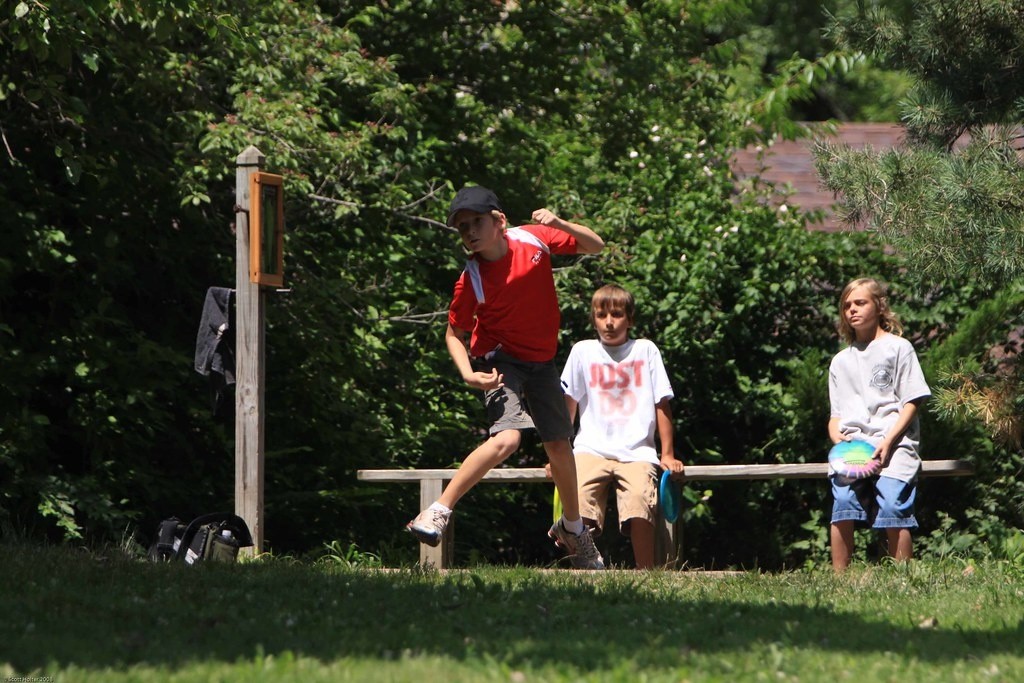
[406,506,454,547]
[547,519,604,570]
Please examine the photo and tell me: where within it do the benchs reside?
[356,461,976,571]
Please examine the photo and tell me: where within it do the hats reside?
[446,186,502,227]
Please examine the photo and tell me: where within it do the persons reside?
[827,277,932,578]
[406,187,604,570]
[546,285,683,570]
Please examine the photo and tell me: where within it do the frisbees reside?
[658,469,682,523]
[828,439,882,480]
